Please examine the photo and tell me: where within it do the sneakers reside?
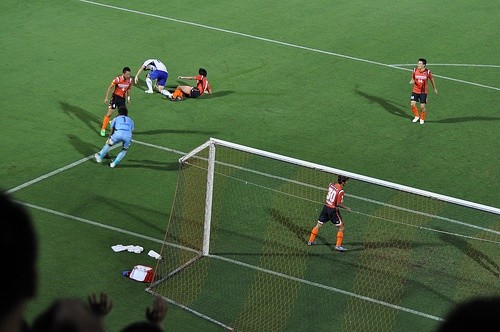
[334,246,349,251]
[308,241,318,245]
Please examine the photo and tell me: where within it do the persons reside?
[165,68,212,102]
[307,175,351,252]
[99,66,133,136]
[134,58,173,99]
[1,190,168,332]
[94,106,134,168]
[408,58,438,125]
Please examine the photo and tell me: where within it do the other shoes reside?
[145,90,153,93]
[110,163,114,167]
[412,116,419,122]
[419,119,424,124]
[101,129,105,136]
[165,95,182,101]
[95,153,101,163]
